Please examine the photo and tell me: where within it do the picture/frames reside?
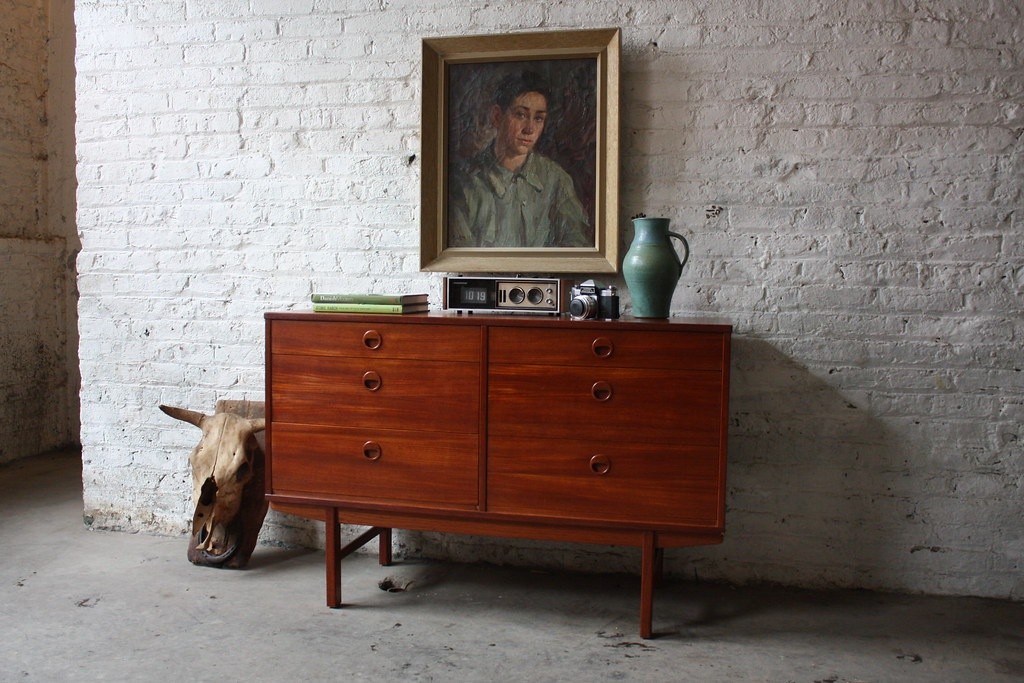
[421,27,621,273]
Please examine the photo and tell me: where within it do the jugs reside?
[621,217,689,319]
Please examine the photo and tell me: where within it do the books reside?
[312,292,429,315]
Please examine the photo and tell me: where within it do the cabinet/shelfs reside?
[264,313,732,639]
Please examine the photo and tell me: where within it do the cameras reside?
[570,280,620,320]
